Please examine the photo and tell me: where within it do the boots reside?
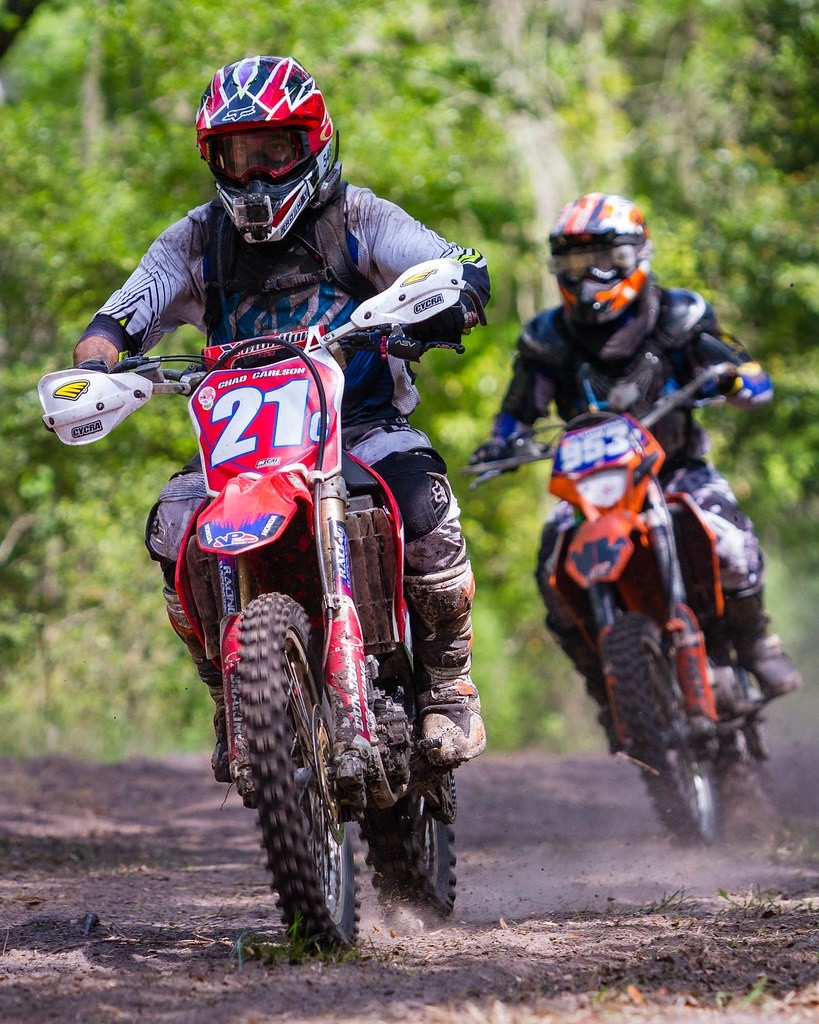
[717,581,802,694]
[401,555,486,763]
[163,575,231,782]
[544,615,622,753]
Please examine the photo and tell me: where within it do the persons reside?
[71,57,491,781]
[469,193,804,753]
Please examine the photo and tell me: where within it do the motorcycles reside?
[33,257,462,953]
[456,329,778,851]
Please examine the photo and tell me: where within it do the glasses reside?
[559,241,634,281]
[220,131,299,182]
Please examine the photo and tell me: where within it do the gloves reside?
[717,365,775,406]
[469,436,518,472]
[75,359,109,373]
[401,301,463,344]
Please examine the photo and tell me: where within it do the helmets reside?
[549,192,652,325]
[194,56,335,243]
